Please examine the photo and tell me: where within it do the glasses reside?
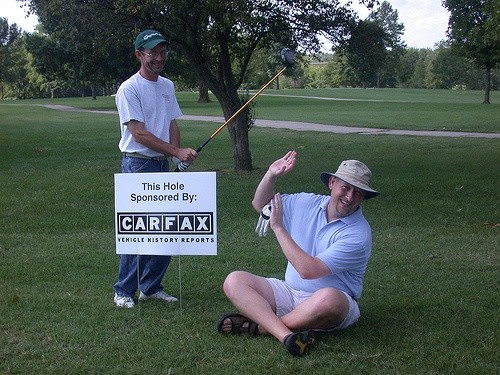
[141,51,169,57]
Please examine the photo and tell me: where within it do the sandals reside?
[216,314,259,338]
[282,329,316,358]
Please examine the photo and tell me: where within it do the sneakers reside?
[114,293,137,309]
[139,291,179,303]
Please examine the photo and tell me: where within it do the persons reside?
[216,151,381,359]
[114,30,198,307]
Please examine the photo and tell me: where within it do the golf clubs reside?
[173,49,296,173]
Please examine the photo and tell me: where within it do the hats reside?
[135,30,171,50]
[321,160,380,199]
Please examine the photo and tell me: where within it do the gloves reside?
[171,157,189,172]
[254,205,271,237]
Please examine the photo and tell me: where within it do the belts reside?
[123,152,165,160]
